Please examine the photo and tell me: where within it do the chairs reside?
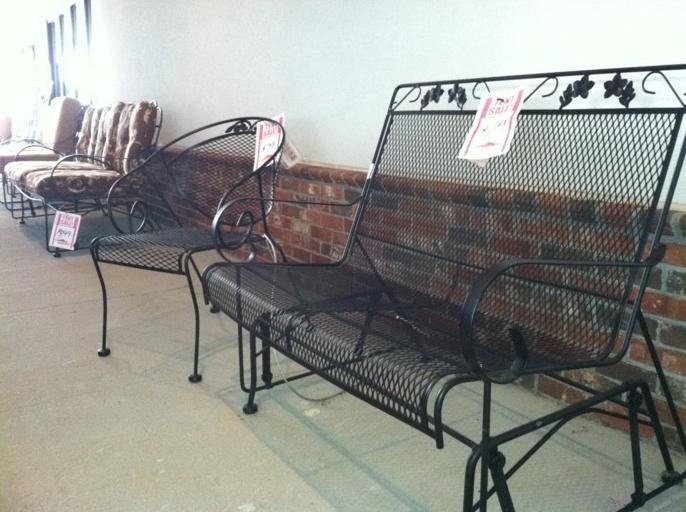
[89,116,314,383]
[0,97,163,257]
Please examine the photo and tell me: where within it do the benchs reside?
[203,64,686,512]
